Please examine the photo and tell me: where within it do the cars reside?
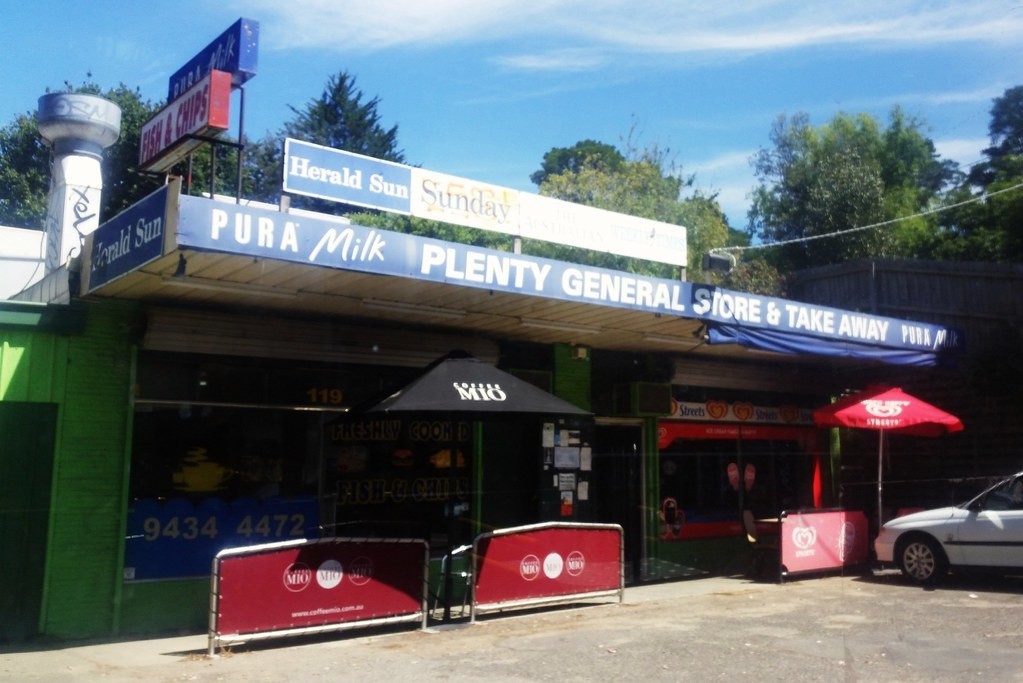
[874,470,1023,584]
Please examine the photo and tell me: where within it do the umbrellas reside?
[814,380,966,537]
[358,348,596,542]
